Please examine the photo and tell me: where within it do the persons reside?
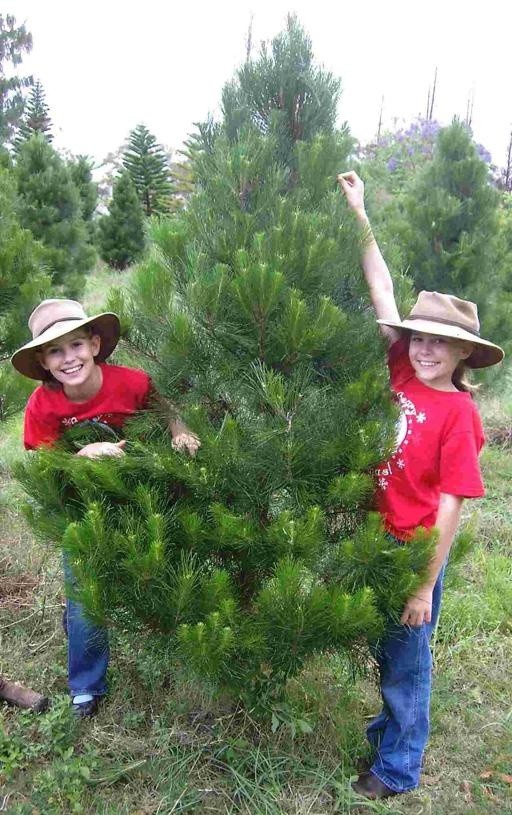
[333,166,505,804]
[11,297,205,727]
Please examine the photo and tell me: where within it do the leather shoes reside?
[350,768,401,803]
[353,749,378,776]
[70,692,101,720]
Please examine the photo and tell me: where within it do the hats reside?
[374,286,506,371]
[9,295,124,385]
